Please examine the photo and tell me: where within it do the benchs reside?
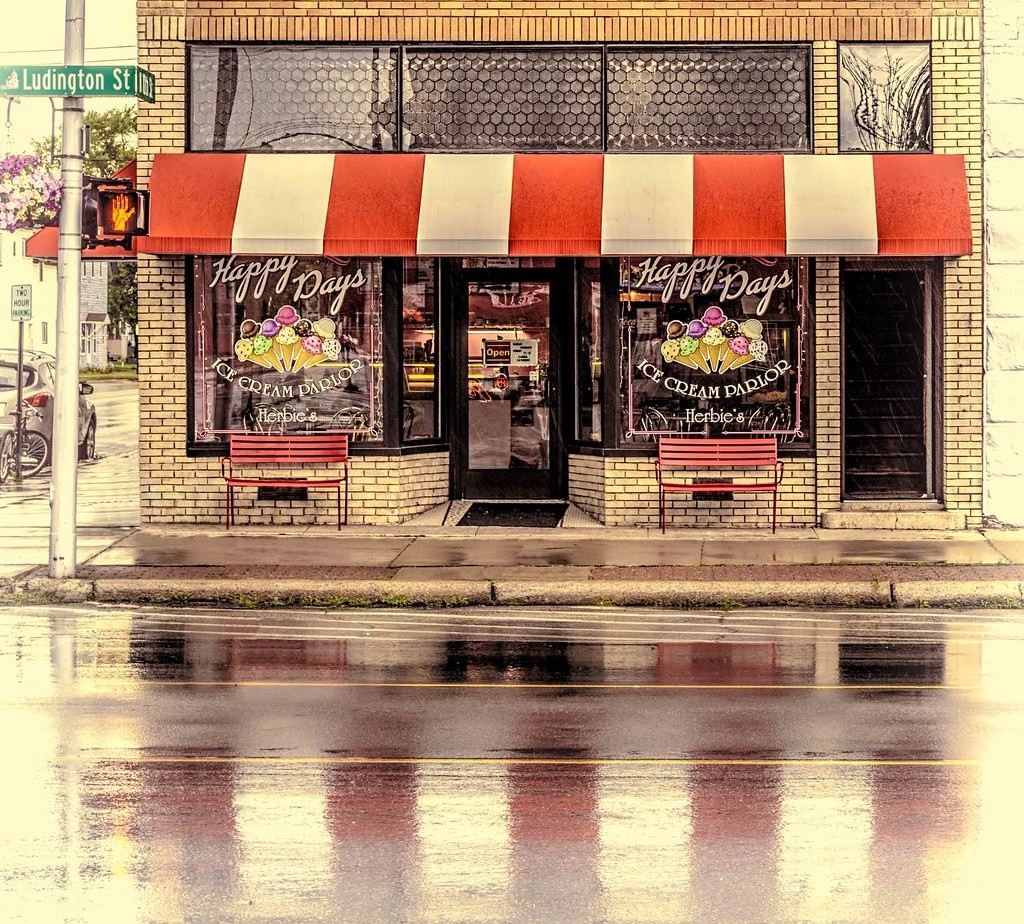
[222,435,352,532]
[655,437,784,534]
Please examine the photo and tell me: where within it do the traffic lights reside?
[100,190,140,238]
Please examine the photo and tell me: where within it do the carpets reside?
[456,502,570,528]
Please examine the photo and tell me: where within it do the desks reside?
[261,416,364,436]
[651,416,767,438]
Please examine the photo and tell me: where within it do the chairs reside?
[243,403,289,437]
[329,401,415,441]
[619,402,791,444]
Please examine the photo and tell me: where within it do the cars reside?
[0,346,97,463]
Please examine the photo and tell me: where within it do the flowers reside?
[0,150,65,234]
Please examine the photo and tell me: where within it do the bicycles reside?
[0,399,50,485]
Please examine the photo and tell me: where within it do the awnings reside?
[139,153,971,260]
[24,159,140,264]
